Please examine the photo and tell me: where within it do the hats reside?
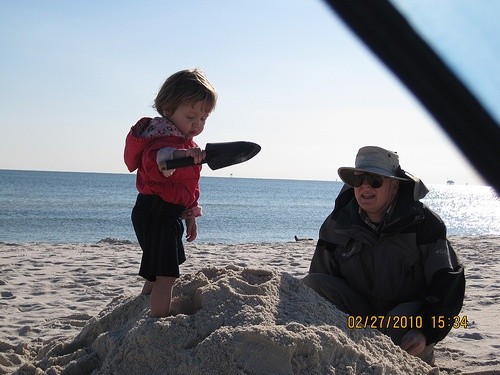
[337,146,412,187]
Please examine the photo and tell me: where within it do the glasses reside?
[351,173,391,189]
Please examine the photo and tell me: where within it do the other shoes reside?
[416,342,436,366]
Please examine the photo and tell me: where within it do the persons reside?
[124,67,219,319]
[301,147,466,365]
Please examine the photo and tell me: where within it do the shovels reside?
[157,141,261,171]
